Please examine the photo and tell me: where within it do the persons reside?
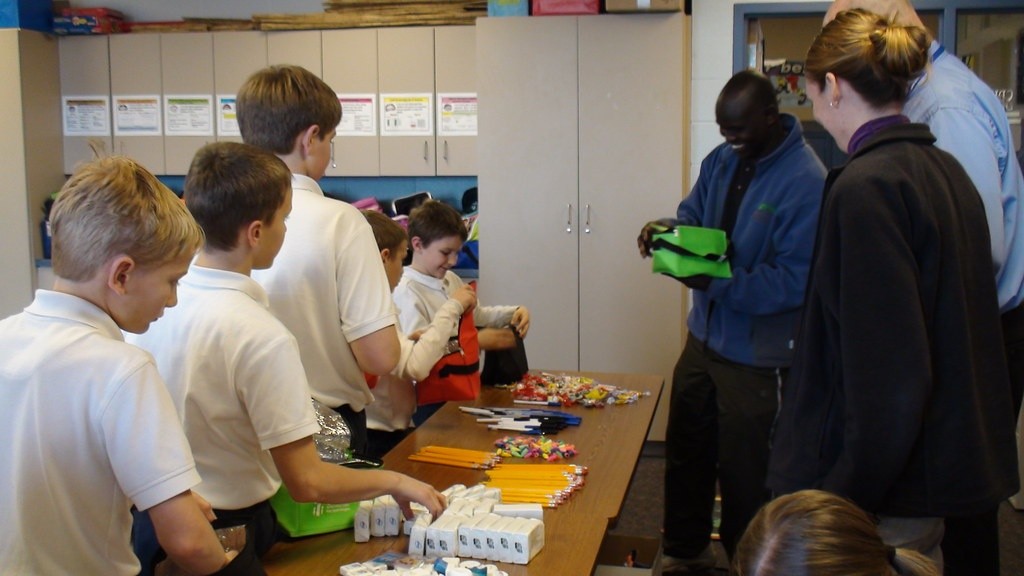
[636,70,826,573]
[768,8,1000,575]
[230,63,401,465]
[390,193,531,423]
[820,0,1023,508]
[351,202,431,471]
[126,141,448,574]
[0,145,236,574]
[732,488,941,575]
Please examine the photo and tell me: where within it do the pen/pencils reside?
[406,407,588,509]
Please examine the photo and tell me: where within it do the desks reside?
[241,368,666,576]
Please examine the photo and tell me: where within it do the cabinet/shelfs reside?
[161,30,269,176]
[477,12,689,447]
[729,0,1024,153]
[265,28,378,179]
[377,24,476,178]
[58,29,167,176]
